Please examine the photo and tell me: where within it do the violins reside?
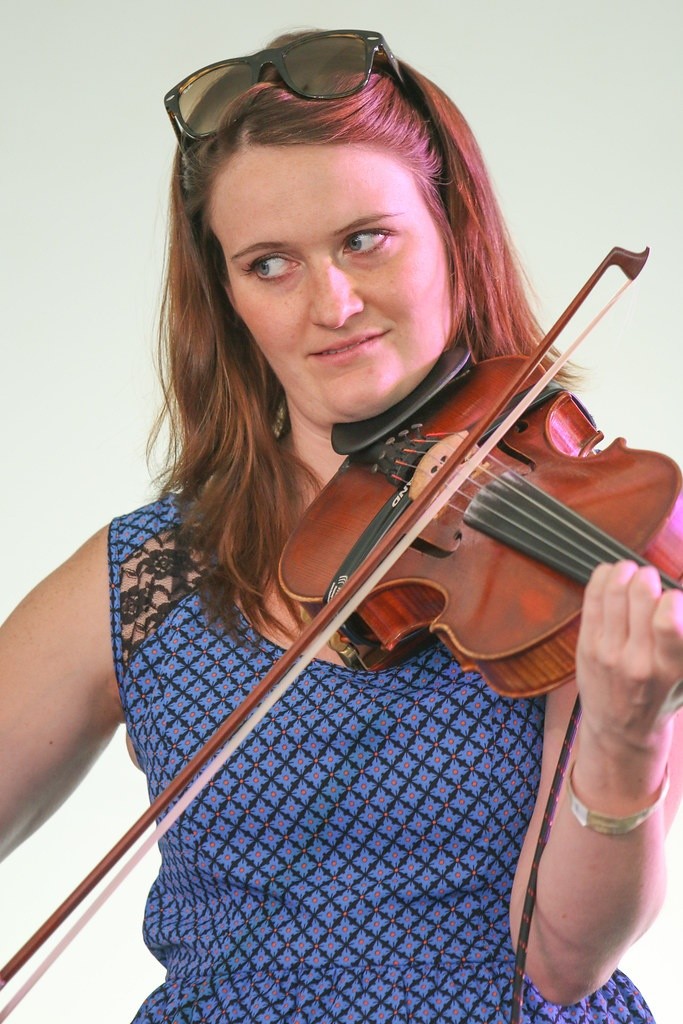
[276,349,682,697]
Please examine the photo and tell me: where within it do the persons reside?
[0,29,683,1024]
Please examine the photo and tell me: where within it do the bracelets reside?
[566,763,670,836]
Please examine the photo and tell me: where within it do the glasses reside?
[164,29,415,154]
